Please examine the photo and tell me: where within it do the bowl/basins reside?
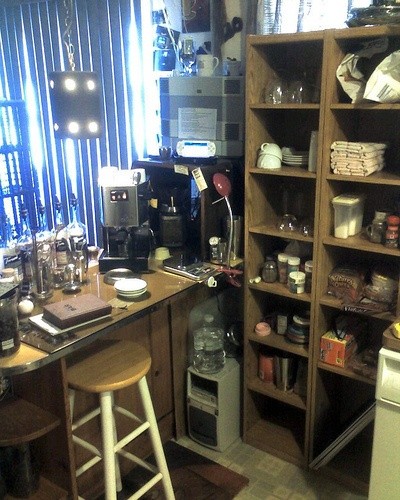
[277,214,299,231]
[297,216,315,237]
[264,79,312,105]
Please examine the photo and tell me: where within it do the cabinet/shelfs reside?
[307,22,400,469]
[134,155,242,261]
[241,22,328,467]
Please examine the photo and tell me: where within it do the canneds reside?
[259,353,273,384]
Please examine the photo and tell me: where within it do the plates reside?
[282,151,311,167]
[114,278,148,299]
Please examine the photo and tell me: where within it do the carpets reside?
[99,441,248,498]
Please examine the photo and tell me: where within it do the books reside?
[43,293,112,330]
[28,313,111,338]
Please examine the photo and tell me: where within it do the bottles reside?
[365,218,387,243]
[52,201,73,288]
[304,260,314,294]
[67,198,89,278]
[384,216,399,249]
[276,253,290,284]
[261,261,279,283]
[331,190,368,239]
[17,208,40,300]
[2,217,29,299]
[286,256,301,288]
[287,271,306,295]
[33,204,57,290]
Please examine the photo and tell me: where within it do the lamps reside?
[212,173,245,287]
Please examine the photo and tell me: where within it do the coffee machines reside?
[97,168,157,274]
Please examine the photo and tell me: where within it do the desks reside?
[0,259,242,500]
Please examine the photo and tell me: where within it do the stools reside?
[64,336,177,500]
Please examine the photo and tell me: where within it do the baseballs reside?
[18,300,34,315]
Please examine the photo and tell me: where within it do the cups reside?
[192,325,226,375]
[210,237,228,266]
[195,54,219,77]
[223,215,243,262]
[257,141,283,160]
[256,155,281,169]
